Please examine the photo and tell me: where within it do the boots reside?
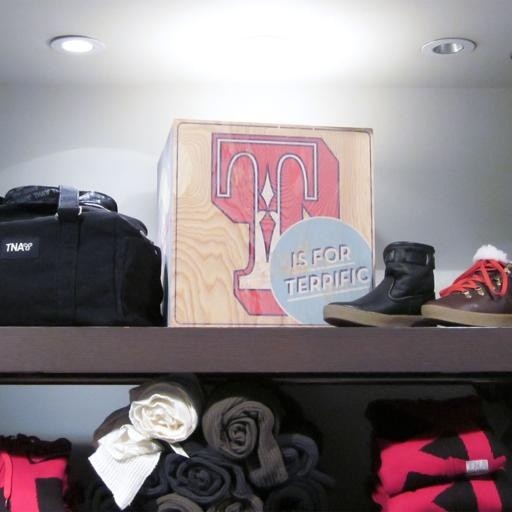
[323,240,439,330]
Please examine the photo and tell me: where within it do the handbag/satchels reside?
[0,433,72,512]
[0,185,167,326]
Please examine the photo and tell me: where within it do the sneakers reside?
[421,259,512,328]
